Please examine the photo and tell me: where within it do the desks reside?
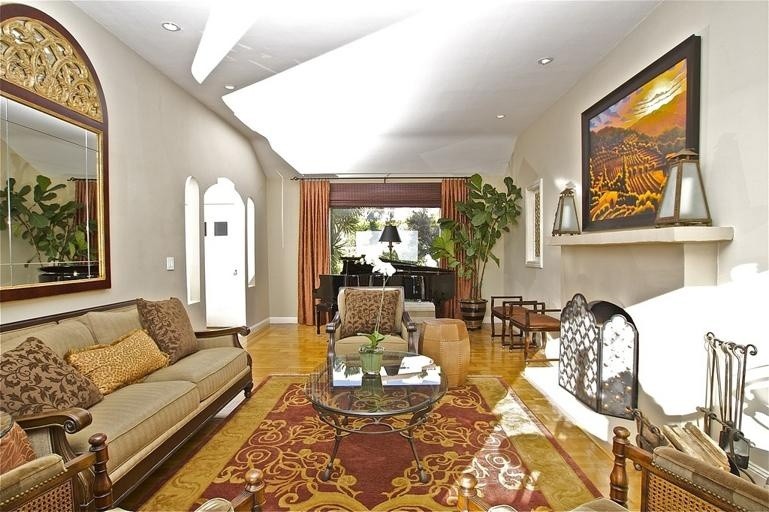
[403,298,436,352]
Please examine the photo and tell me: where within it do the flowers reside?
[353,253,398,353]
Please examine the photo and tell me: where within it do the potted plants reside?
[426,171,523,330]
[0,171,99,285]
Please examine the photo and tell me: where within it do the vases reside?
[359,349,385,375]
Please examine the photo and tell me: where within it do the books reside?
[380,364,441,386]
[333,366,362,386]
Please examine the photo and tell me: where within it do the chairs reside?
[326,285,418,395]
[0,432,269,511]
[448,424,768,511]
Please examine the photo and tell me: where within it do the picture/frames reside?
[579,32,702,232]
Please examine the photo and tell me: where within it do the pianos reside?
[312,258,457,334]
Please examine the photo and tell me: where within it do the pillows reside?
[63,326,171,397]
[0,410,39,478]
[0,336,106,417]
[133,296,201,367]
[337,288,401,339]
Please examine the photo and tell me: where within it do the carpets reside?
[131,374,608,511]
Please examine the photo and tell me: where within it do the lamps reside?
[379,223,403,262]
[652,146,715,228]
[551,187,582,237]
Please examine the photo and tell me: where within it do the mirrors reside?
[0,2,111,304]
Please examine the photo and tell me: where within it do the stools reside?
[491,294,536,346]
[315,301,339,334]
[506,301,565,364]
[418,317,473,389]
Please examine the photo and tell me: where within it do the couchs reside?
[0,295,254,511]
